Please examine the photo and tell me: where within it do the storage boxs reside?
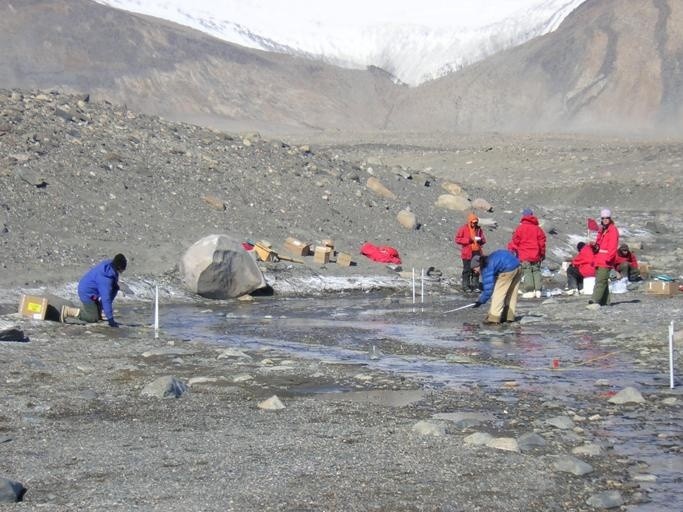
[18,294,56,321]
[253,243,278,262]
[643,280,678,295]
[284,237,352,267]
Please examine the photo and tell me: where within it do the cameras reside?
[592,244,599,253]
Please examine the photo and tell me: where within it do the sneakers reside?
[586,303,607,311]
[564,288,579,296]
[482,317,514,327]
[522,290,542,299]
[464,288,481,293]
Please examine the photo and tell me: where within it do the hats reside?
[620,244,629,252]
[470,255,481,270]
[523,209,533,216]
[601,209,612,218]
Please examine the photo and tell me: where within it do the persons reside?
[454,213,486,294]
[59,253,127,327]
[470,249,523,326]
[615,244,644,283]
[506,208,546,298]
[564,242,596,295]
[585,208,619,309]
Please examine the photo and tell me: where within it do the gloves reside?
[472,302,481,308]
